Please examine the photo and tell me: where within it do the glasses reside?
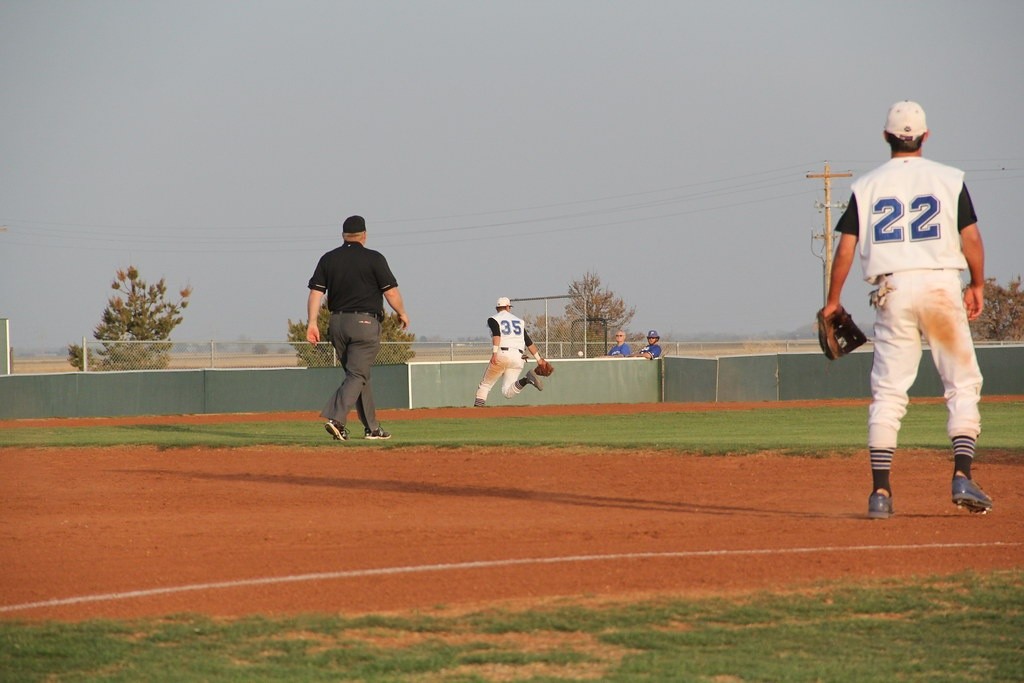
[616,335,623,337]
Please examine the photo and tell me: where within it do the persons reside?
[607,330,631,357]
[821,99,994,519]
[306,215,409,441]
[473,296,546,407]
[635,329,662,360]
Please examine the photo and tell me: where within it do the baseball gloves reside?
[816,304,869,361]
[534,358,554,377]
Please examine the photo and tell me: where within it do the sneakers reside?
[325,419,350,441]
[364,428,392,439]
[526,369,544,391]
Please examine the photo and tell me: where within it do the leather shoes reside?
[868,493,895,519]
[952,476,992,514]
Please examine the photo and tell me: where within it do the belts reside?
[332,311,382,323]
[501,347,524,354]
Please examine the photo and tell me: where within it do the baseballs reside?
[577,350,584,357]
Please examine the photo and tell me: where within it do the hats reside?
[885,100,927,141]
[496,297,514,307]
[343,216,366,233]
[647,330,658,338]
